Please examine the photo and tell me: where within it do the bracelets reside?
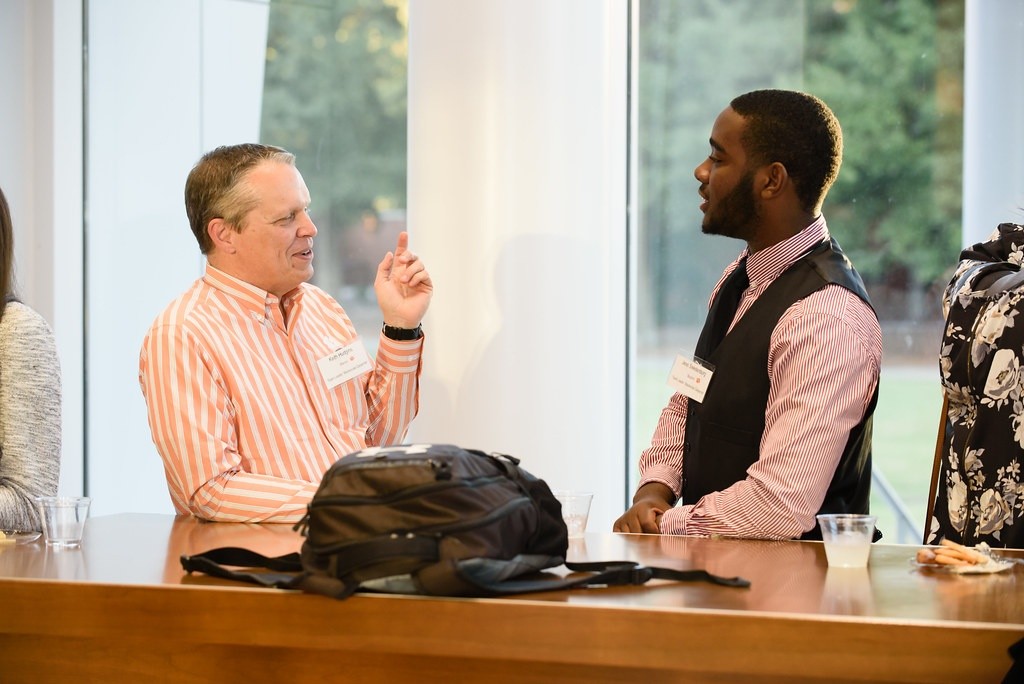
[382,322,422,340]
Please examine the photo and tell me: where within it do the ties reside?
[694,256,751,362]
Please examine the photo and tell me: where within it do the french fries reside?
[935,539,989,565]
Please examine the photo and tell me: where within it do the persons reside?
[138,144,433,523]
[612,89,882,542]
[924,223,1024,550]
[0,189,61,532]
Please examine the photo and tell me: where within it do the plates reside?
[952,558,1018,573]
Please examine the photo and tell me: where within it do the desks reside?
[0,513,1024,684]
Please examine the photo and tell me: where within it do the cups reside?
[817,514,876,570]
[36,497,95,549]
[553,495,593,534]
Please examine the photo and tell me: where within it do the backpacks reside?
[181,445,750,597]
